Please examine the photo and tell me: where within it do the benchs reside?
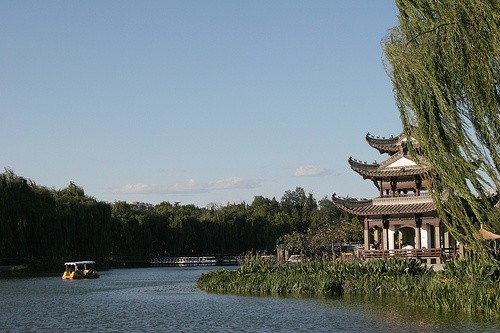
[362,246,455,257]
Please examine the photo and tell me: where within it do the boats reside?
[62,261,100,279]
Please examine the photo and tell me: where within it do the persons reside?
[76,267,82,276]
[402,242,413,256]
[88,266,93,274]
